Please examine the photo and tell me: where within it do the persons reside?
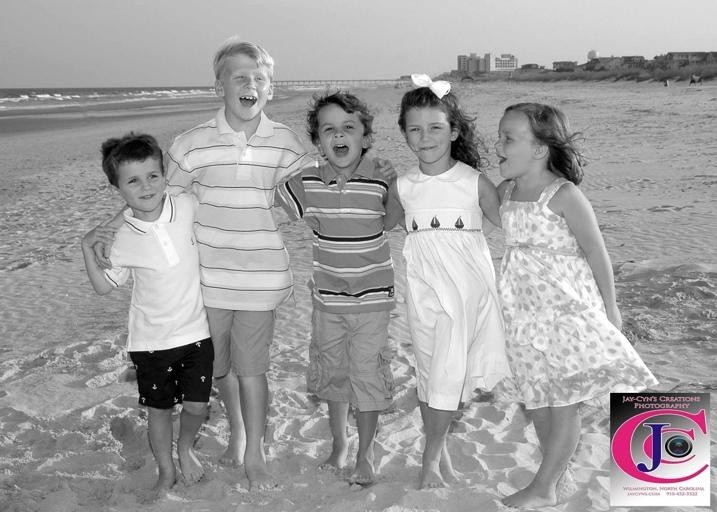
[79,130,215,496]
[274,88,407,491]
[697,72,705,85]
[689,72,695,86]
[90,37,398,496]
[383,71,503,493]
[492,100,660,511]
